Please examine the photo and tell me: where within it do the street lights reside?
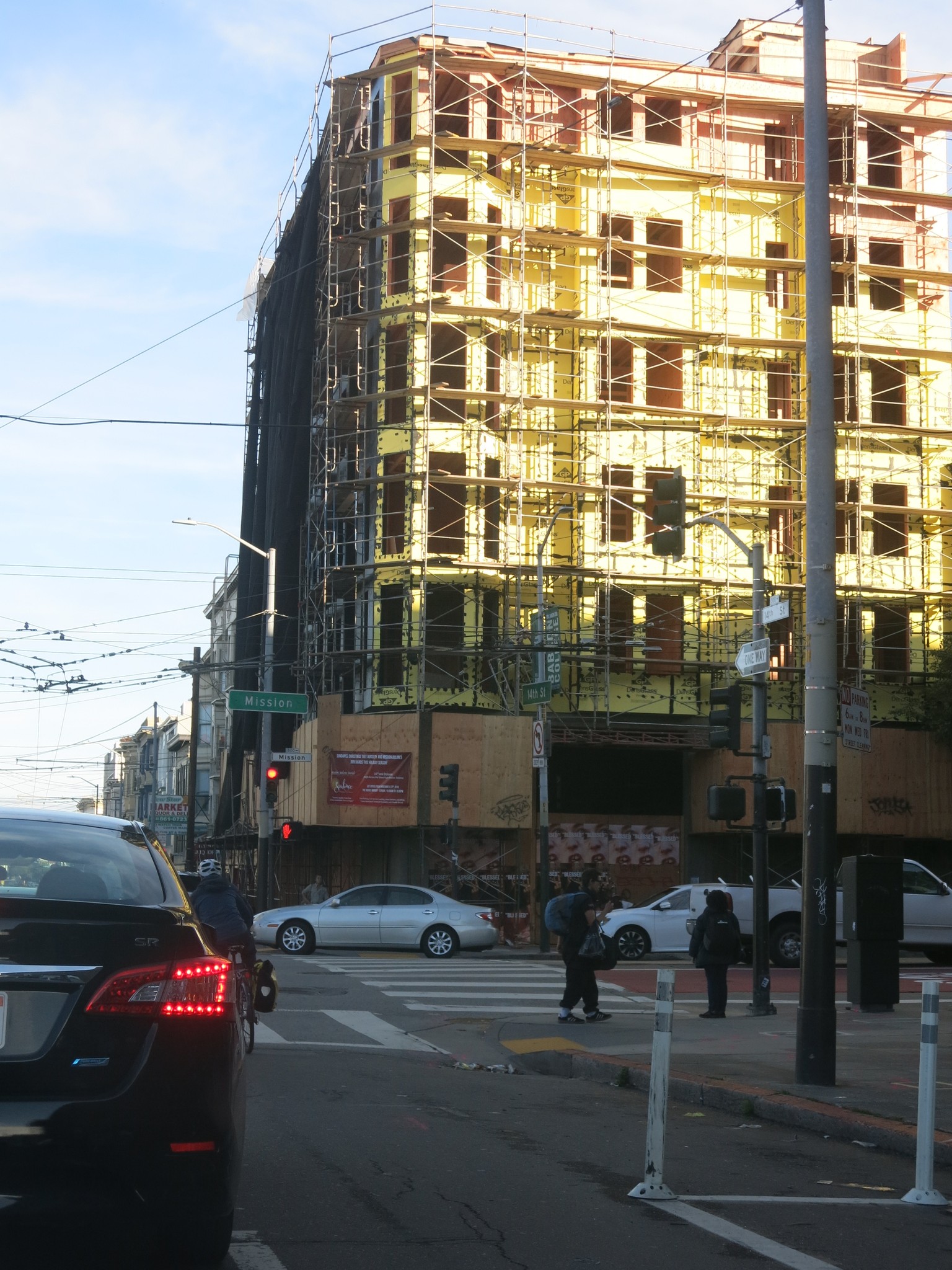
[66,773,99,815]
[83,739,124,818]
[171,514,277,913]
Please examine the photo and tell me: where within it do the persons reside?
[689,890,748,1018]
[189,857,258,997]
[301,874,330,906]
[4,861,99,890]
[555,869,616,1025]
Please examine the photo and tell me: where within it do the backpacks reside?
[702,908,735,953]
[546,891,591,935]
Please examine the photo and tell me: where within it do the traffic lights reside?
[265,766,278,803]
[439,761,460,803]
[708,679,741,749]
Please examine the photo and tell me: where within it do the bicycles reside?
[223,924,260,1056]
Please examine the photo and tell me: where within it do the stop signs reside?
[281,821,303,843]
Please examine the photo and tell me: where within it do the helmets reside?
[197,858,223,877]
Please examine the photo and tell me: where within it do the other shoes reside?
[699,1009,718,1018]
[717,1010,726,1018]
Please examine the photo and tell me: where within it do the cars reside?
[249,883,498,960]
[0,801,244,1268]
[592,883,717,960]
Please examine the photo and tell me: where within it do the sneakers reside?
[558,1013,586,1023]
[586,1012,613,1022]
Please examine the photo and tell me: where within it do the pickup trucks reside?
[686,854,952,968]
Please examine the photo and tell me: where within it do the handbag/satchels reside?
[250,960,278,1012]
[592,919,618,972]
[579,919,605,956]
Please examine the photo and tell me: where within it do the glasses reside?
[594,879,603,884]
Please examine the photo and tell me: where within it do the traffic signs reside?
[840,683,874,753]
[734,636,771,678]
